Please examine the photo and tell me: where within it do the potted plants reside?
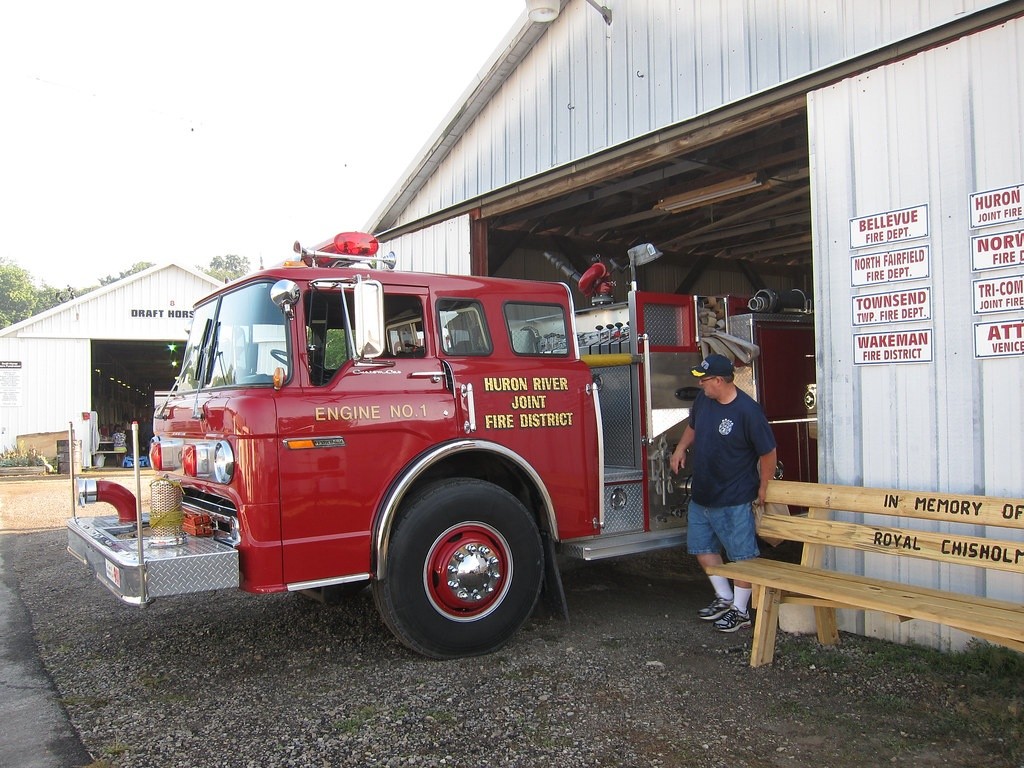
[0,444,45,475]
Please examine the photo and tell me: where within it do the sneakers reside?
[713,605,752,632]
[696,593,734,621]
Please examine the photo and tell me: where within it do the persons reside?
[112,425,127,467]
[670,352,777,632]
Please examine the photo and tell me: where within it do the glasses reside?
[700,377,723,383]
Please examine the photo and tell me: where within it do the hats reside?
[691,354,733,377]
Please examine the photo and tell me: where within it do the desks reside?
[98,441,114,443]
[95,451,124,467]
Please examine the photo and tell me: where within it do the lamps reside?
[525,0,611,25]
[652,172,770,215]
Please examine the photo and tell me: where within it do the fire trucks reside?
[63,231,818,661]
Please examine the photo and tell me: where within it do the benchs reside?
[706,480,1023,669]
